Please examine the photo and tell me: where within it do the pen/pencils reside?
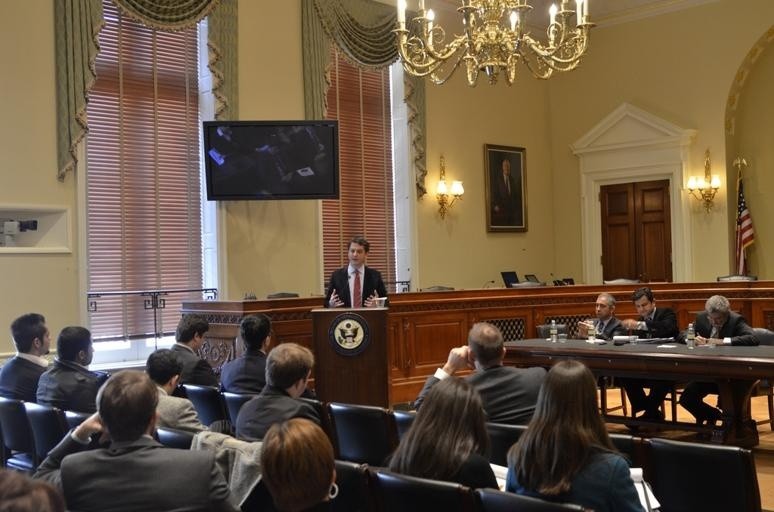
[695,332,706,343]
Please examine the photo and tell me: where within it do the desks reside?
[311,307,388,414]
[503,339,774,447]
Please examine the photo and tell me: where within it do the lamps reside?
[687,149,721,214]
[391,0,598,87]
[436,156,464,220]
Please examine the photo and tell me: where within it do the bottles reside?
[550,320,557,343]
[687,323,695,350]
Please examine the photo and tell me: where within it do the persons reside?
[614,287,679,429]
[577,293,628,341]
[0,314,53,404]
[233,344,324,444]
[146,349,210,435]
[258,416,379,512]
[172,312,220,386]
[495,155,521,226]
[218,315,319,397]
[29,370,242,512]
[0,470,65,512]
[387,379,502,497]
[680,295,761,436]
[35,326,110,414]
[323,239,390,308]
[416,322,550,429]
[503,358,645,511]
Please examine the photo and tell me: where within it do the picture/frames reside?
[525,275,539,283]
[483,143,528,232]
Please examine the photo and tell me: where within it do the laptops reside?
[500,271,521,289]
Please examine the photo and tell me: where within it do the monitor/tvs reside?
[524,275,541,283]
[202,119,340,201]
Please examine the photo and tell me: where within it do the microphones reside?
[482,280,495,289]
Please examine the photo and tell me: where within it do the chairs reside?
[326,402,390,466]
[300,397,329,425]
[747,328,774,431]
[642,437,773,512]
[63,412,100,451]
[420,286,455,291]
[390,410,416,442]
[0,397,38,472]
[267,292,299,299]
[178,384,230,432]
[485,423,529,467]
[609,433,642,467]
[474,488,594,512]
[603,279,640,283]
[156,426,194,449]
[511,281,547,287]
[536,324,571,339]
[24,402,67,457]
[220,392,257,435]
[630,383,703,425]
[192,429,272,512]
[717,275,758,281]
[334,460,368,504]
[598,328,632,416]
[363,469,473,512]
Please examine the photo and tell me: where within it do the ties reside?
[354,268,361,310]
[598,320,604,332]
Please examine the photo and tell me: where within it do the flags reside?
[731,156,755,277]
